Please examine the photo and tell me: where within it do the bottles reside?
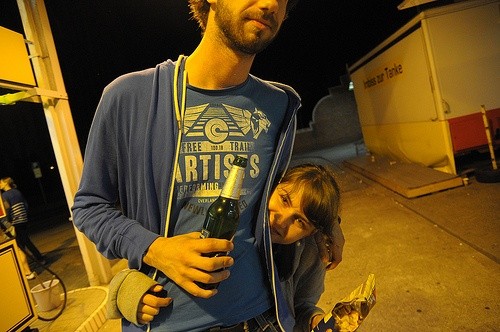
[193,156,249,290]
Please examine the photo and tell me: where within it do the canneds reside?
[371,155,375,162]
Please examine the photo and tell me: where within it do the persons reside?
[0,217,37,281]
[107,163,340,332]
[0,177,47,267]
[71,0,345,332]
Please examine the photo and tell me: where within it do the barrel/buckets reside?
[31,279,62,311]
